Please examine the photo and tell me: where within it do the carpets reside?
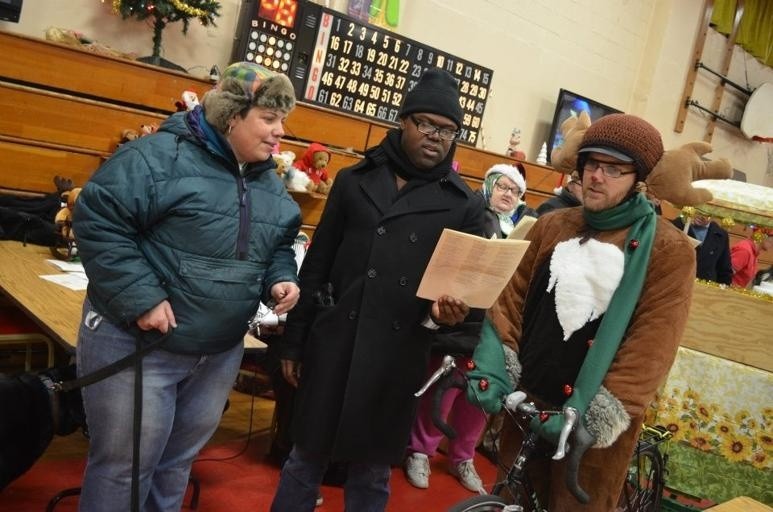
[0,403,513,510]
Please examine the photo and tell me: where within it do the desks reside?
[1,236,272,379]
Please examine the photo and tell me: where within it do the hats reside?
[577,144,634,163]
[205,61,295,131]
[395,67,464,129]
[484,162,527,199]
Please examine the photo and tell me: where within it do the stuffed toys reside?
[269,142,333,196]
[54,87,201,257]
[467,115,698,512]
[506,128,525,161]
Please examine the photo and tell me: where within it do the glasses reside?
[583,157,638,179]
[411,115,460,141]
[495,182,523,198]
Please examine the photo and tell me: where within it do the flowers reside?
[98,1,222,39]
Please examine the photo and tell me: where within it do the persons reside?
[72,61,302,512]
[221,228,311,474]
[537,174,773,298]
[405,163,536,493]
[270,68,484,512]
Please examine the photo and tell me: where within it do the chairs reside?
[0,318,58,378]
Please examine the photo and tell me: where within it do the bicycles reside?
[411,353,672,510]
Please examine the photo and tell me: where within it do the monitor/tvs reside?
[546,88,625,166]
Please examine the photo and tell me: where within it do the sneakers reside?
[404,452,430,489]
[442,457,483,492]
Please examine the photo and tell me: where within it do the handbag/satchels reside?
[0,192,60,244]
